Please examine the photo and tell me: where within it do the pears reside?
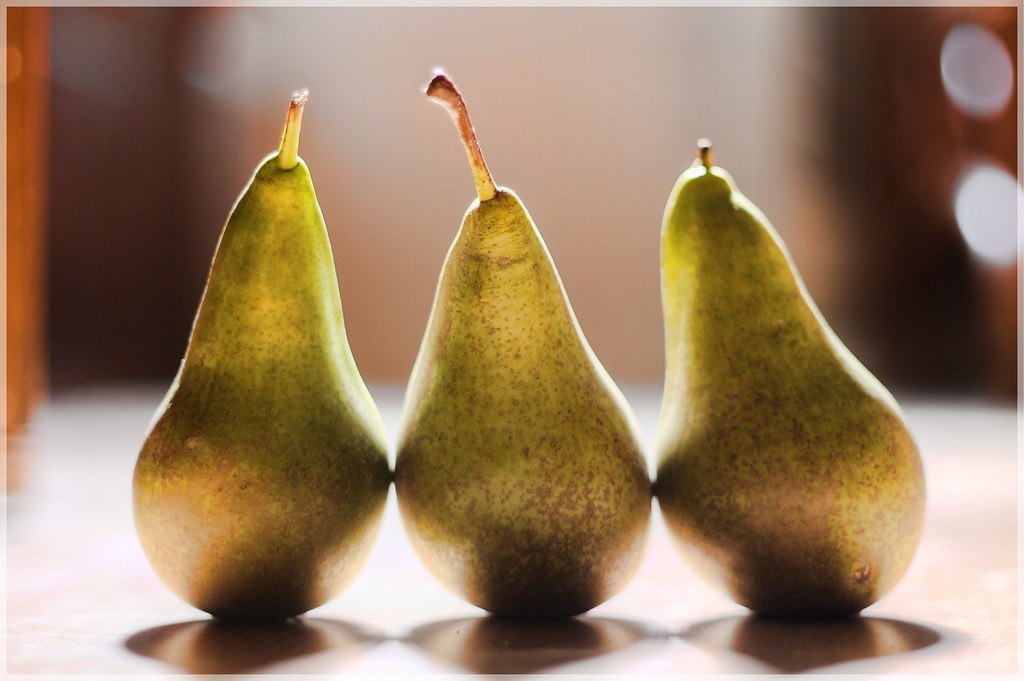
[393,72,654,618]
[132,86,394,622]
[653,137,928,622]
